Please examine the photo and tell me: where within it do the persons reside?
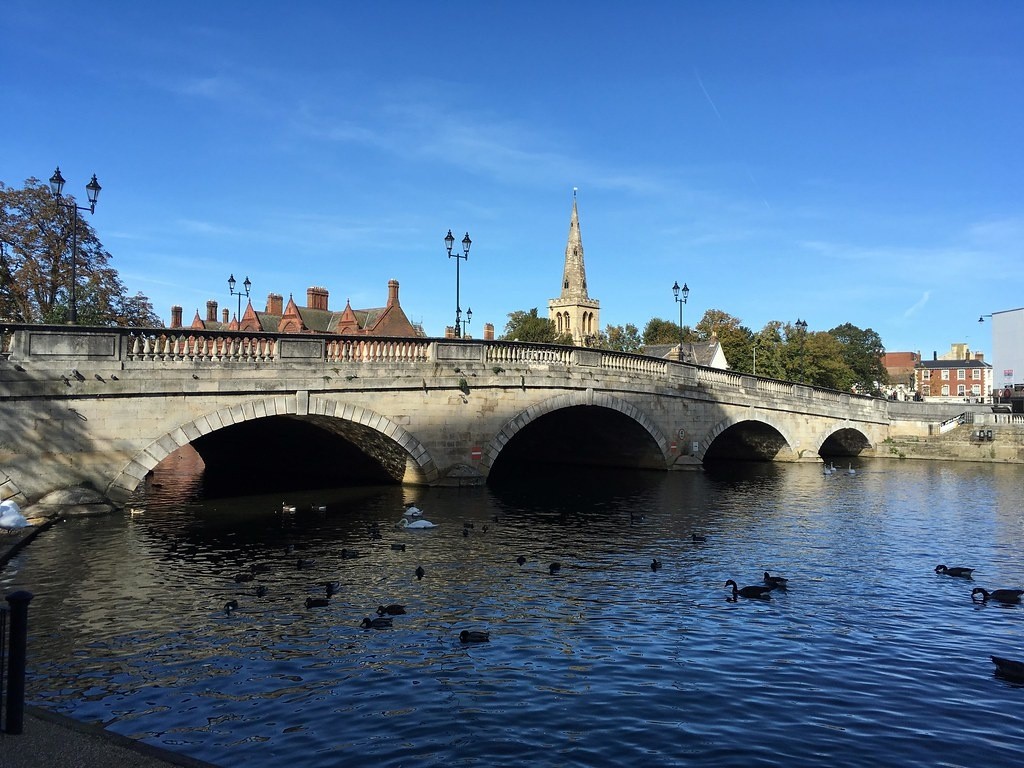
[893,391,897,400]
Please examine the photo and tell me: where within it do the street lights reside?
[226,274,251,331]
[48,166,102,325]
[795,319,809,383]
[445,231,471,337]
[457,306,473,340]
[672,280,689,360]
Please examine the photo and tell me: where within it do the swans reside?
[823,461,855,475]
[0,500,33,534]
[394,505,440,529]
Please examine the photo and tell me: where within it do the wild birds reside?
[462,515,498,536]
[459,631,490,642]
[129,507,147,515]
[630,512,645,520]
[690,533,706,541]
[650,558,662,571]
[990,655,1024,684]
[309,502,328,510]
[548,563,561,573]
[360,604,406,629]
[222,549,359,615]
[366,522,382,539]
[934,565,975,578]
[517,556,526,566]
[971,588,1024,604]
[282,501,296,510]
[391,544,406,550]
[415,566,425,580]
[725,571,789,597]
